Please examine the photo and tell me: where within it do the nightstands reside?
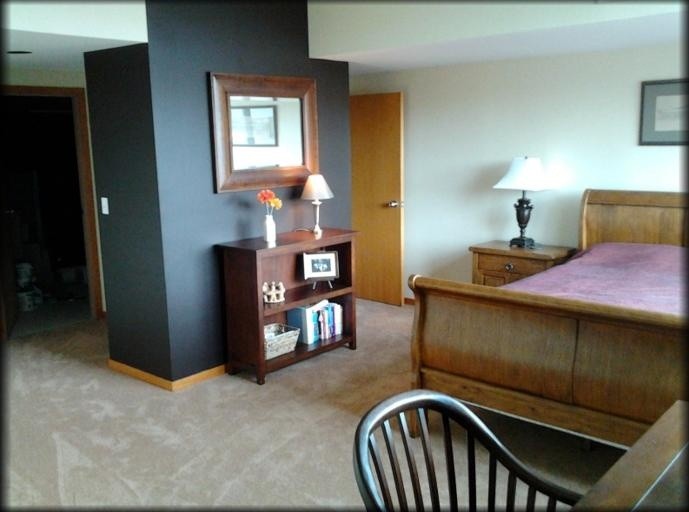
[468,240,574,286]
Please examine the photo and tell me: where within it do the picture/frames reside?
[303,251,340,288]
[638,77,689,146]
[210,72,319,193]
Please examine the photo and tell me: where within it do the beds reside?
[408,188,689,449]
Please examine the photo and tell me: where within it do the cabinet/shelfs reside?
[213,228,359,386]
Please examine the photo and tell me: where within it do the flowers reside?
[257,190,282,215]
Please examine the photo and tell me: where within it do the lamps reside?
[301,174,334,234]
[493,155,556,249]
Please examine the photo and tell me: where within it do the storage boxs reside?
[287,299,327,344]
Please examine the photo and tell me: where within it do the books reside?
[286,300,343,344]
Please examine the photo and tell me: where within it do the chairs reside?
[352,389,584,512]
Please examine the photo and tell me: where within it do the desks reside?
[570,400,689,511]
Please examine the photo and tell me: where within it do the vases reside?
[264,215,276,248]
